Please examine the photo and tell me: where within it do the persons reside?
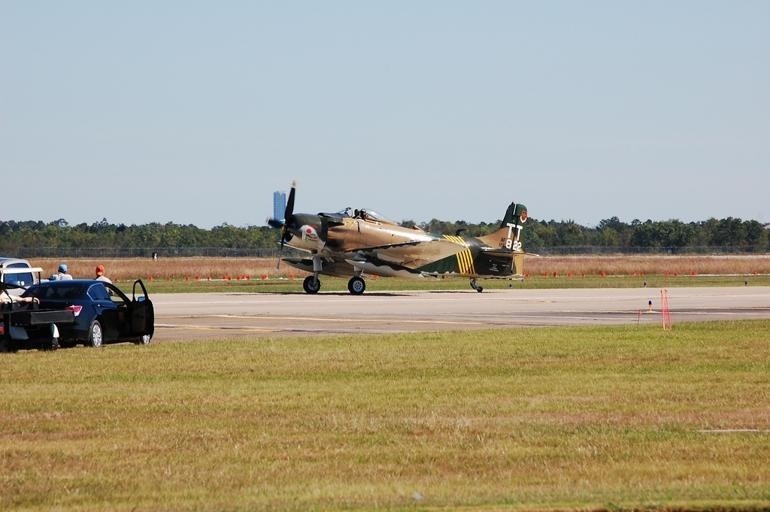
[353,209,359,219]
[56,264,73,280]
[95,265,113,296]
[359,208,366,219]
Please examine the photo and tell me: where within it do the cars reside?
[0,257,156,353]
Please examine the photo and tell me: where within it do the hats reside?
[59,265,67,272]
[96,265,104,276]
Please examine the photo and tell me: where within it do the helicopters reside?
[266,180,529,297]
